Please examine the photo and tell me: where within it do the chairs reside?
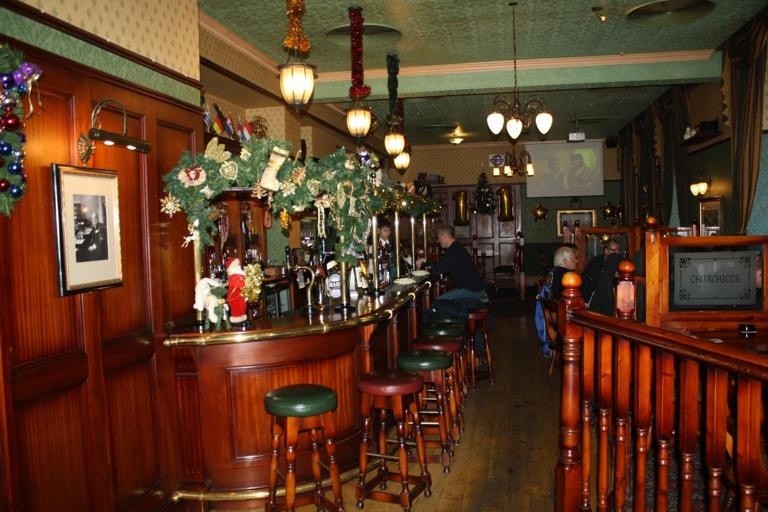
[536,290,563,387]
[405,238,522,299]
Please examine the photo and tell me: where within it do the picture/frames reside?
[698,194,725,234]
[52,162,125,299]
[556,209,597,238]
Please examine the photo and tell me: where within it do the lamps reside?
[77,97,154,164]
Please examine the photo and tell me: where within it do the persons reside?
[428,225,489,300]
[566,154,596,195]
[376,219,412,277]
[545,238,628,315]
[542,159,564,195]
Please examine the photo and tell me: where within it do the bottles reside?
[214,103,236,136]
[324,249,341,300]
[348,243,396,294]
[358,290,385,315]
[206,249,287,283]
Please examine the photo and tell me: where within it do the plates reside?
[392,278,416,284]
[412,270,430,276]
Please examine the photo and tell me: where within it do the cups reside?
[739,323,757,337]
